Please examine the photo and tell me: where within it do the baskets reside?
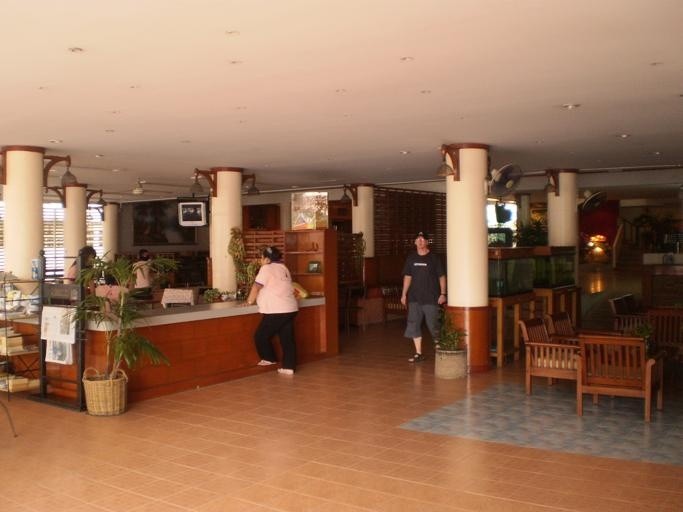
[83,367,128,415]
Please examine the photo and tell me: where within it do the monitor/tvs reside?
[178,201,208,227]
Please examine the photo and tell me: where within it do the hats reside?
[413,231,429,239]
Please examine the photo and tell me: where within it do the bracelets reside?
[441,294,447,296]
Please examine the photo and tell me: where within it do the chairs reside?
[516,309,683,424]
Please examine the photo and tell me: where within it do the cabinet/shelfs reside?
[282,229,325,300]
[1,246,48,404]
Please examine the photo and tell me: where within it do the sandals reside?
[409,354,424,362]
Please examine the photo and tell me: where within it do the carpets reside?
[394,379,682,467]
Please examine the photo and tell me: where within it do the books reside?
[0,327,40,392]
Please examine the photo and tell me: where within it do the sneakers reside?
[278,368,294,374]
[257,359,277,366]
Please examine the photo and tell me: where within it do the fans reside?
[109,181,173,197]
[486,162,522,198]
[577,188,608,213]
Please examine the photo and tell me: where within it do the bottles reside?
[31,259,41,281]
[168,279,176,288]
[185,279,194,288]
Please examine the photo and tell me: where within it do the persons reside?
[64,246,96,284]
[132,249,153,294]
[401,231,447,363]
[240,246,299,374]
[95,267,129,306]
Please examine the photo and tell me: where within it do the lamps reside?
[188,173,205,194]
[247,177,260,195]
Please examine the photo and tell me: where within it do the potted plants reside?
[77,255,186,416]
[430,312,470,379]
